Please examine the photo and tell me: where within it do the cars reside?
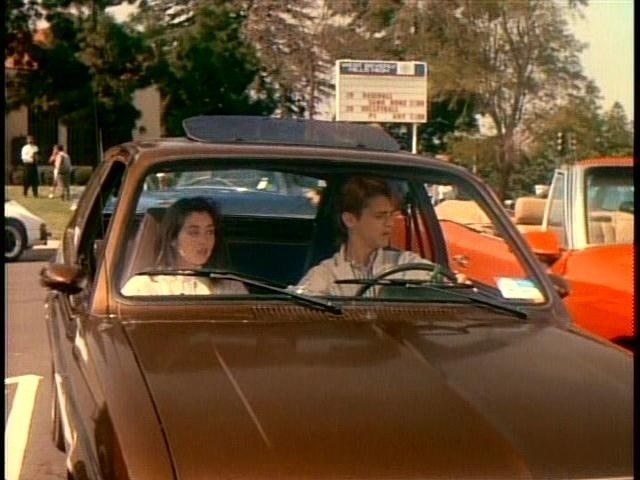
[4,199,46,261]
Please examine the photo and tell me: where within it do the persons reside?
[48,144,73,200]
[120,196,249,296]
[297,172,472,296]
[20,135,40,197]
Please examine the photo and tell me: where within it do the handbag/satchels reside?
[59,164,65,174]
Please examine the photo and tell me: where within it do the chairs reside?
[514,196,635,250]
[115,208,229,291]
[305,188,368,268]
